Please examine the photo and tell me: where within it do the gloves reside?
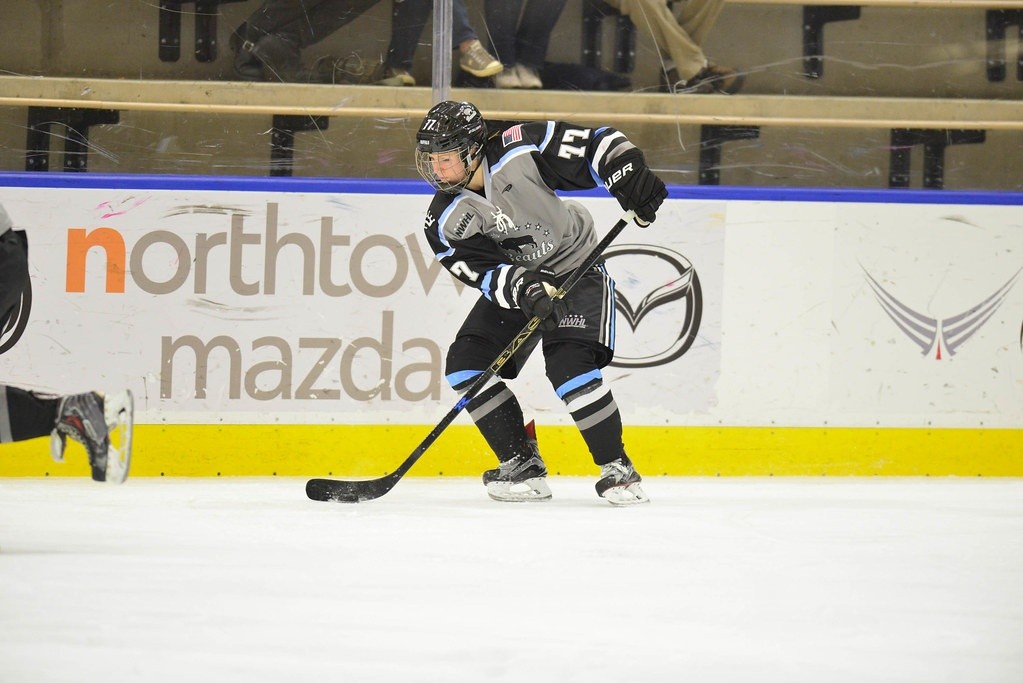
[602,150,669,224]
[511,264,571,332]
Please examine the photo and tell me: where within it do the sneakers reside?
[374,65,415,86]
[50,389,134,484]
[595,442,649,506]
[482,419,552,502]
[458,40,503,78]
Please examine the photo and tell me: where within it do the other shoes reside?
[516,62,542,89]
[491,64,521,88]
[251,32,330,83]
[696,62,746,95]
[662,67,714,94]
[229,22,264,81]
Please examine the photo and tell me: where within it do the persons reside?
[220,0,746,95]
[0,204,134,484]
[414,101,669,506]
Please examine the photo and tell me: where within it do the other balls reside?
[336,493,359,502]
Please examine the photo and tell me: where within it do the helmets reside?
[415,101,488,196]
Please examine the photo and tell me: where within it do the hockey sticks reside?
[305,209,634,502]
